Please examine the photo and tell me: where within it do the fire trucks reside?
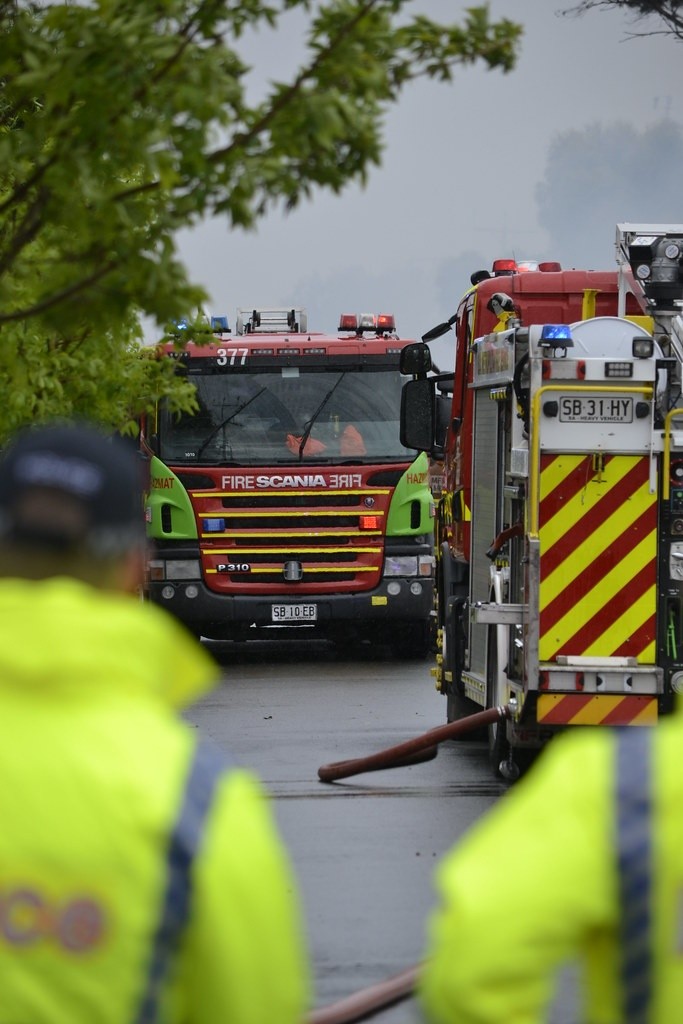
[131,308,438,665]
[398,222,683,786]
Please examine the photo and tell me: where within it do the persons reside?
[416,686,682,1024]
[0,421,312,1024]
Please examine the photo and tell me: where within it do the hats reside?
[0,426,148,563]
